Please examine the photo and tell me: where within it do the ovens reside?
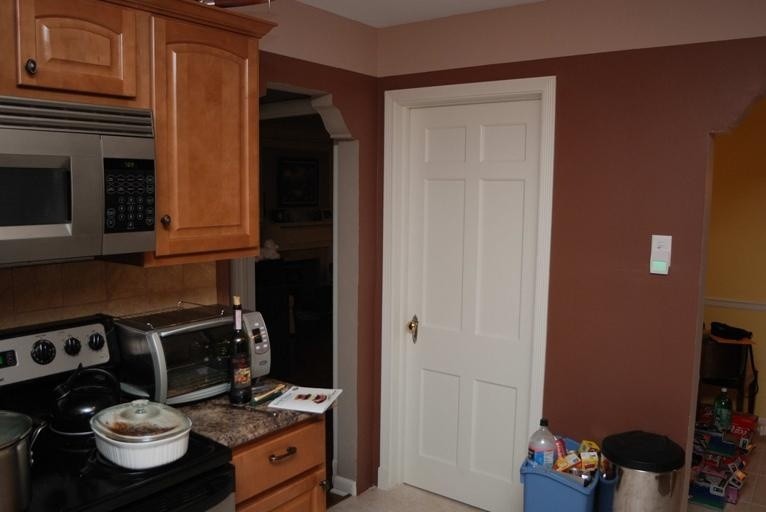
[107,463,238,512]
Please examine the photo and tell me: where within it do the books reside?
[267,385,344,414]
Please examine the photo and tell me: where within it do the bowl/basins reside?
[87,400,192,472]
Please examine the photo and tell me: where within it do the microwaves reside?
[114,302,270,406]
[0,130,156,265]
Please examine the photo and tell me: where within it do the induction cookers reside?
[0,383,233,512]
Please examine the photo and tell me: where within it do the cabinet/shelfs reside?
[150,13,264,267]
[231,413,328,512]
[0,0,153,112]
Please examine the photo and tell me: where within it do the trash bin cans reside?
[520,437,599,512]
[601,430,686,511]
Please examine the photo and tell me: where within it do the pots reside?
[0,410,50,512]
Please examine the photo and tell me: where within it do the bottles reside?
[311,206,331,221]
[526,418,559,472]
[551,433,566,460]
[230,295,251,404]
[712,389,734,434]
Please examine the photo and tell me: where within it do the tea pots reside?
[48,366,123,433]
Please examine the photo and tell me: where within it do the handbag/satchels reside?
[700,321,752,388]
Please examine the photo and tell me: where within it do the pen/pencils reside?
[249,384,286,406]
